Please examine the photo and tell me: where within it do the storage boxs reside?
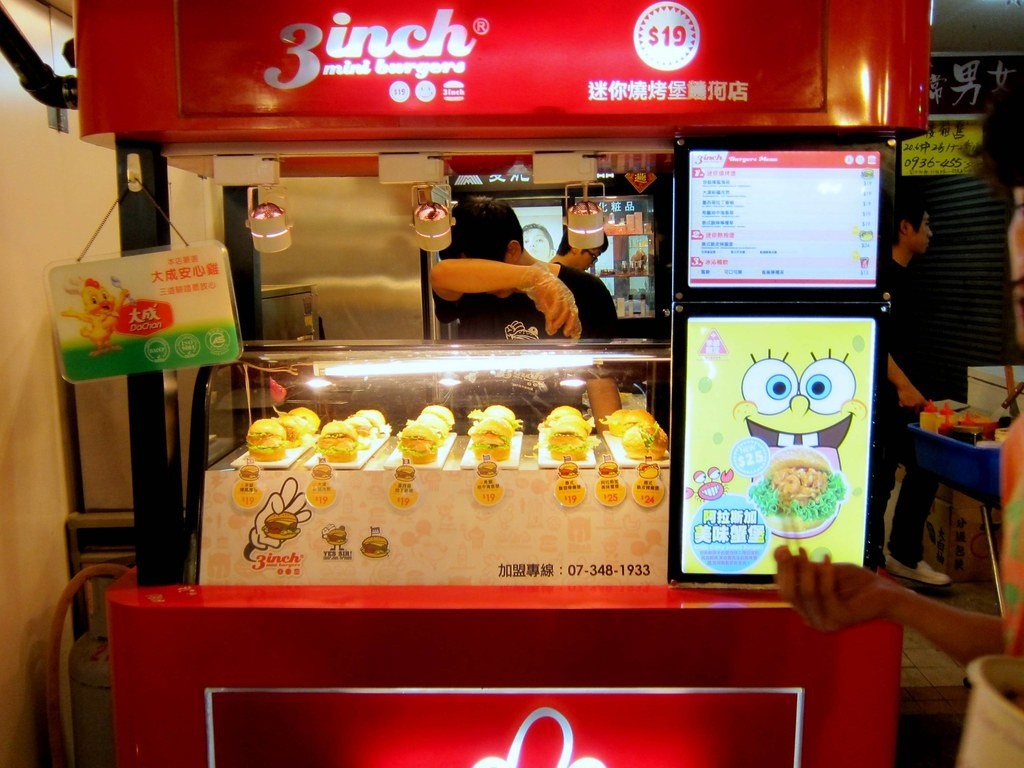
[907,420,1001,507]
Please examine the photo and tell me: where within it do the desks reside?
[890,450,1008,617]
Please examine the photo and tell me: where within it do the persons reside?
[427,196,623,437]
[868,192,951,586]
[774,408,1024,666]
[550,226,609,273]
[522,224,556,262]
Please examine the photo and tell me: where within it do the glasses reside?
[586,248,598,265]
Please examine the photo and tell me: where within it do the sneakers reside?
[884,554,950,586]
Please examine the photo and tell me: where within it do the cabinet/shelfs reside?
[574,197,656,319]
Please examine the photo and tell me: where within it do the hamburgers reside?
[245,405,669,467]
[748,444,845,534]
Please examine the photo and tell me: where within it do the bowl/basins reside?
[955,654,1024,768]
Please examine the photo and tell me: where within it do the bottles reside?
[635,263,638,274]
[938,414,955,436]
[623,263,627,274]
[920,400,938,434]
[958,411,975,428]
[939,403,958,436]
[628,294,634,317]
[641,294,646,317]
[631,263,634,275]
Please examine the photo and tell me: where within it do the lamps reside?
[409,183,460,251]
[566,181,608,249]
[244,184,296,254]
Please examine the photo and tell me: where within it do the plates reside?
[304,431,391,470]
[461,431,523,469]
[538,430,596,468]
[231,434,322,470]
[602,431,670,468]
[384,432,457,470]
